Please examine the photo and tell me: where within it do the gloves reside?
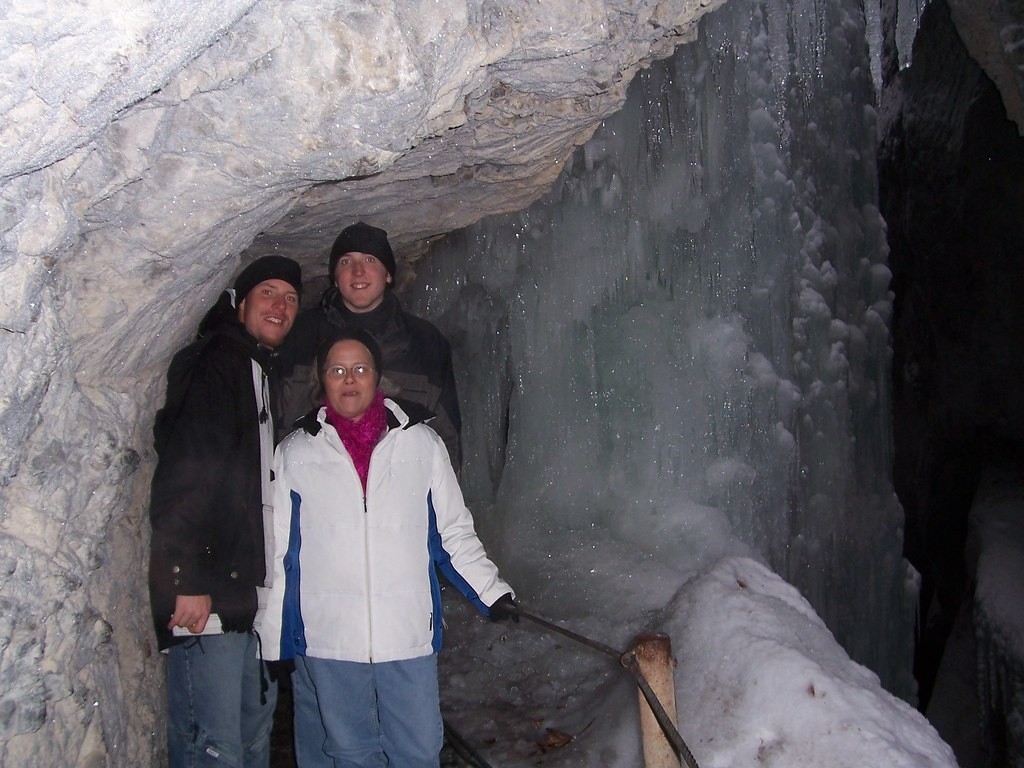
[489,592,520,624]
[265,656,296,689]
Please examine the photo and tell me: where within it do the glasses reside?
[325,364,373,380]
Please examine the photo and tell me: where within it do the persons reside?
[148,255,307,768]
[255,327,519,768]
[270,219,461,767]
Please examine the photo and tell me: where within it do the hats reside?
[234,254,302,308]
[328,223,396,289]
